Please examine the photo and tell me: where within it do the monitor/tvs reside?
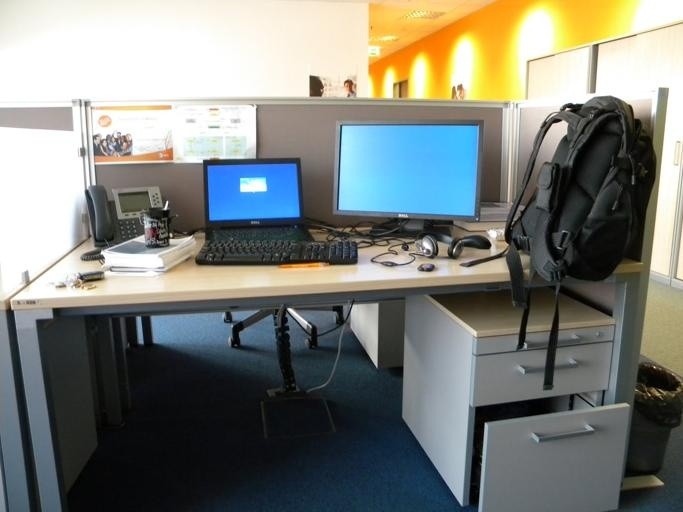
[331,120,485,245]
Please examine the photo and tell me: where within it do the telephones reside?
[85,184,163,247]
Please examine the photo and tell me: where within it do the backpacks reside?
[504,96,655,285]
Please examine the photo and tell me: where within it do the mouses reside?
[461,235,492,249]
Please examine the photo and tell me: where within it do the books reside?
[102,234,196,278]
[451,202,524,241]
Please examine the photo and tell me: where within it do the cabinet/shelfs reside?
[524,20,682,290]
[399,292,634,512]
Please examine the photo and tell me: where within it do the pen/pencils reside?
[278,262,328,268]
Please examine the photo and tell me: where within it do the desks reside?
[9,226,643,512]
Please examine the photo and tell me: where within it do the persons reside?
[93,131,133,157]
[343,79,356,98]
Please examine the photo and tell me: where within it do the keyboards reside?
[196,240,358,265]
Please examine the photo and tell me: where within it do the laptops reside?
[202,158,315,242]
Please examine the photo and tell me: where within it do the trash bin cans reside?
[625,362,683,475]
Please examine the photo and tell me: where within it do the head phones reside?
[414,231,464,258]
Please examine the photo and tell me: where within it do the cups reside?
[139,207,171,248]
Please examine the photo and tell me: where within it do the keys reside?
[53,270,104,290]
